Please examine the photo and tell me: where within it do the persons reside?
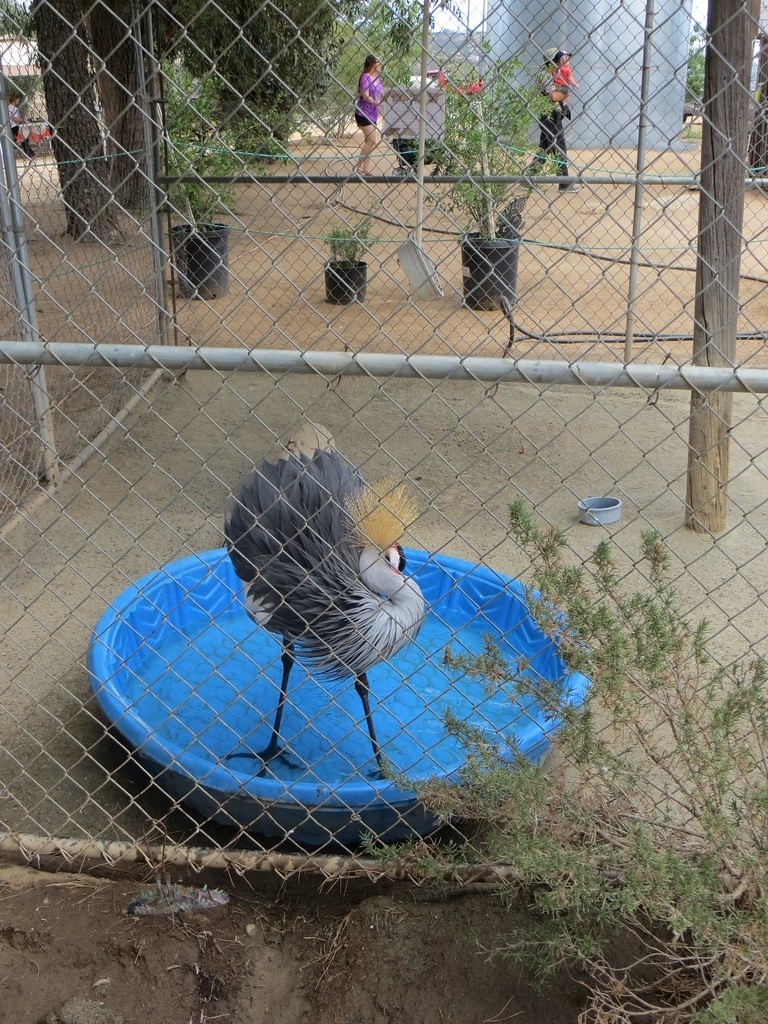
[8,92,36,157]
[552,50,579,120]
[518,48,581,193]
[354,54,384,176]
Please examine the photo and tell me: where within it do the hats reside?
[554,50,572,60]
[540,48,559,67]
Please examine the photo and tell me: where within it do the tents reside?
[0,33,42,76]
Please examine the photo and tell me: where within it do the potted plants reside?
[415,38,566,310]
[323,193,379,305]
[145,57,292,304]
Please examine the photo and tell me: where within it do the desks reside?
[17,122,52,150]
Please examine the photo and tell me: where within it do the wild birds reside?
[216,422,424,781]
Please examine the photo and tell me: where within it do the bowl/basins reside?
[576,497,622,526]
[396,235,445,303]
[92,538,593,848]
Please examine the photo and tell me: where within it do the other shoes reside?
[520,184,541,191]
[563,104,571,120]
[363,174,375,177]
[559,186,581,192]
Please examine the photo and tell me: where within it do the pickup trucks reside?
[426,69,486,98]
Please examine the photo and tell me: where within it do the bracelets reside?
[573,84,577,89]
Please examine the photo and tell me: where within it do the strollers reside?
[390,138,458,177]
